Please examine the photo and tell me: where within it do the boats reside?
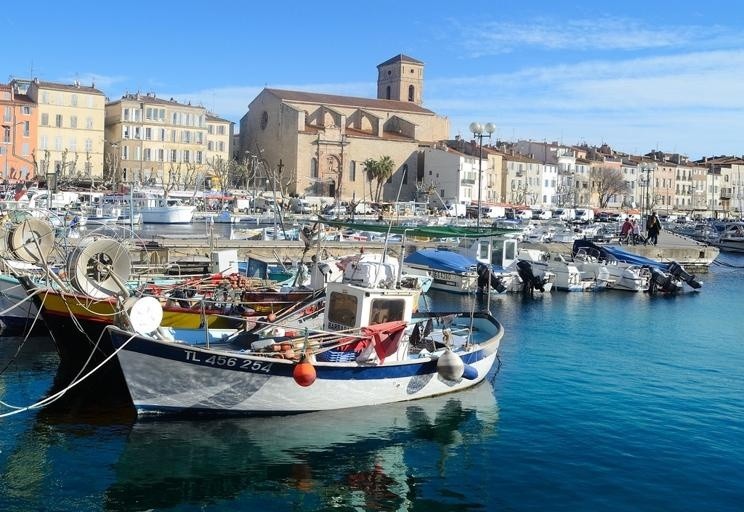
[2,149,706,417]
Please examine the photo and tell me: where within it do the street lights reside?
[469,119,495,235]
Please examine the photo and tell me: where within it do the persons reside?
[643,215,660,246]
[631,220,642,246]
[617,218,634,246]
[646,211,663,244]
